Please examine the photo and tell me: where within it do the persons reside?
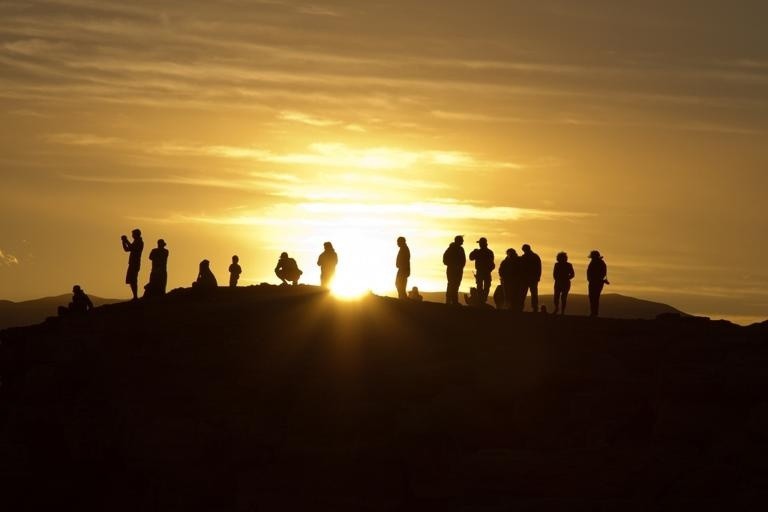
[411,287,423,302]
[317,243,337,286]
[587,251,607,317]
[275,252,304,285]
[438,235,546,313]
[228,255,243,286]
[192,260,218,288]
[395,237,411,299]
[121,228,144,299]
[148,239,169,300]
[58,285,94,316]
[553,253,575,315]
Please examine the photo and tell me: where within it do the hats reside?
[476,237,487,244]
[587,250,603,259]
